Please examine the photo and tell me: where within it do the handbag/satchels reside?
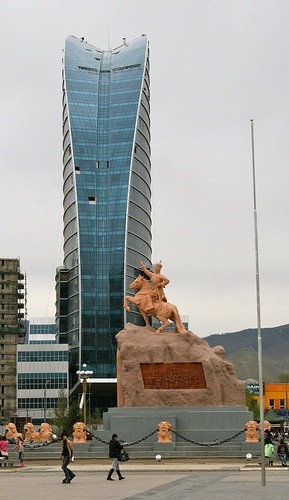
[1,450,8,456]
[119,447,129,462]
[69,455,74,462]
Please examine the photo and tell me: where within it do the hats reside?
[63,436,67,440]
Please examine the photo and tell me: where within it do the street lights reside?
[76,364,94,426]
[44,381,49,423]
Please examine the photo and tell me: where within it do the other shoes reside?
[17,462,26,467]
[62,477,70,484]
[70,473,75,481]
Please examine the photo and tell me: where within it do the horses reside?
[123,274,188,335]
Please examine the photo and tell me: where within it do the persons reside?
[262,430,289,467]
[106,434,125,481]
[140,261,169,314]
[0,434,8,467]
[17,436,24,467]
[60,434,76,484]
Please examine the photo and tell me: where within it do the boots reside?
[107,468,114,480]
[116,470,125,480]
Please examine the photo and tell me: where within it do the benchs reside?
[0,459,20,468]
[258,458,289,466]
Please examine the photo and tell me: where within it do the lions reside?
[244,421,271,443]
[157,422,173,442]
[72,422,88,444]
[5,423,53,445]
